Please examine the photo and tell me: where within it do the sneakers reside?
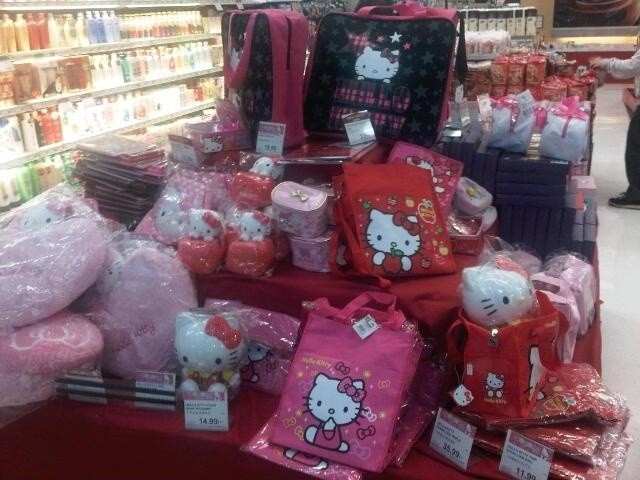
[609,192,640,209]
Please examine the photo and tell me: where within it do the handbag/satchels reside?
[249,291,449,480]
[303,6,468,147]
[331,162,457,288]
[446,291,570,416]
[218,8,306,148]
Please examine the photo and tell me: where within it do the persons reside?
[590,48,640,210]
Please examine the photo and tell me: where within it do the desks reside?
[0,144,599,480]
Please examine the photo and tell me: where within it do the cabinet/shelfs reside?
[0,0,224,214]
[453,6,538,61]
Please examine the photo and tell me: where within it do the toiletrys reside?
[0,10,225,213]
